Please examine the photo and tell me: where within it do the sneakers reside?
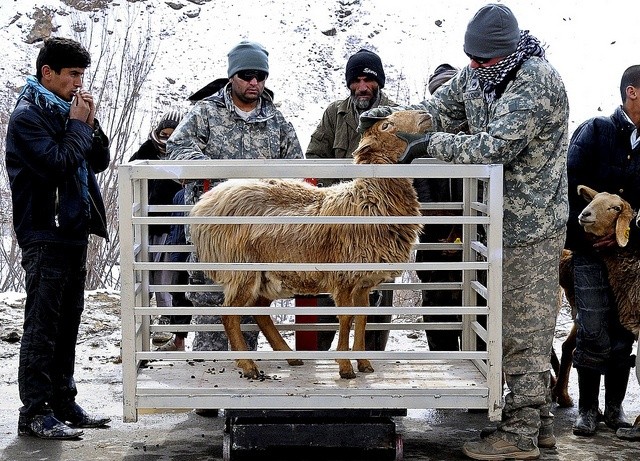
[153,339,185,351]
[153,315,173,342]
[479,419,557,447]
[463,431,540,461]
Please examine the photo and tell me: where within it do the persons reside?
[357,4,571,459]
[309,49,400,350]
[128,109,183,343]
[165,40,304,418]
[7,36,111,439]
[566,66,640,435]
[411,65,486,353]
[155,178,193,352]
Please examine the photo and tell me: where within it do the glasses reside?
[463,52,492,64]
[237,70,268,82]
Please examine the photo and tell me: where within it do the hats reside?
[345,48,385,88]
[157,109,185,134]
[228,40,269,79]
[464,4,521,58]
[428,63,460,94]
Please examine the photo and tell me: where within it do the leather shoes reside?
[18,414,84,440]
[55,403,111,428]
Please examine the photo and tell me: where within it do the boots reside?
[604,363,632,428]
[573,365,601,434]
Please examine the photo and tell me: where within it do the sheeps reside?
[576,184,639,339]
[188,108,433,380]
